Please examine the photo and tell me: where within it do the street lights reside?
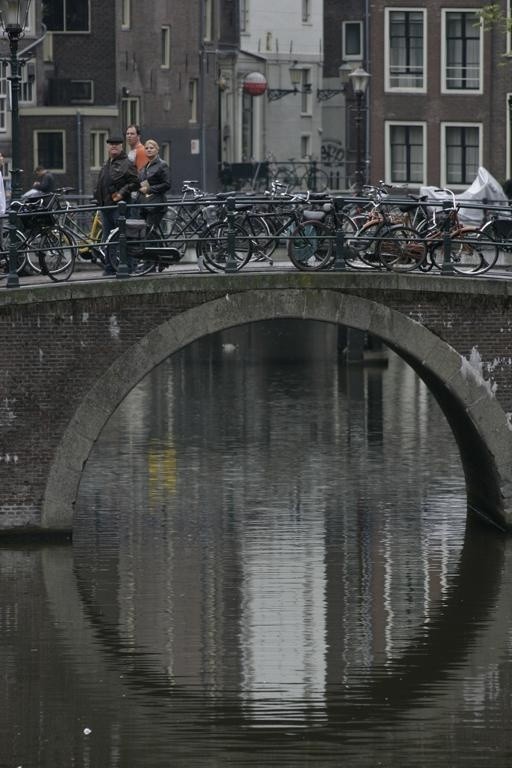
[0,0,33,289]
[346,64,372,197]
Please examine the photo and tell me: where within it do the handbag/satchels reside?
[142,193,163,211]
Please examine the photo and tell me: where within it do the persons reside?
[0,152,7,215]
[96,136,141,277]
[137,139,173,274]
[34,167,56,206]
[125,123,148,176]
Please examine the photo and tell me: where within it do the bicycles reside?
[274,154,330,193]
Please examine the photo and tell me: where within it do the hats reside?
[107,136,124,144]
